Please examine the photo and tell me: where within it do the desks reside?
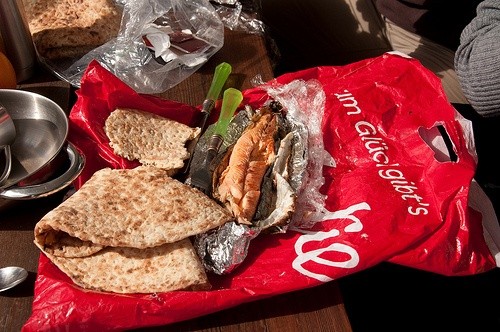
[0,12,352,332]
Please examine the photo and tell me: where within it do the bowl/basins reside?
[0,140,86,229]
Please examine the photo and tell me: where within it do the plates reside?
[0,89,68,189]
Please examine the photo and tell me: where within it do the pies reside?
[105,107,202,171]
[16,0,124,59]
[32,165,234,294]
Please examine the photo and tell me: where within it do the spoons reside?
[0,266,28,292]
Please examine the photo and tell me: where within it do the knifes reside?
[175,62,232,174]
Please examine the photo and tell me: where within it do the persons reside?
[453,0,500,117]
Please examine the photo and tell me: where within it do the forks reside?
[189,86,244,195]
